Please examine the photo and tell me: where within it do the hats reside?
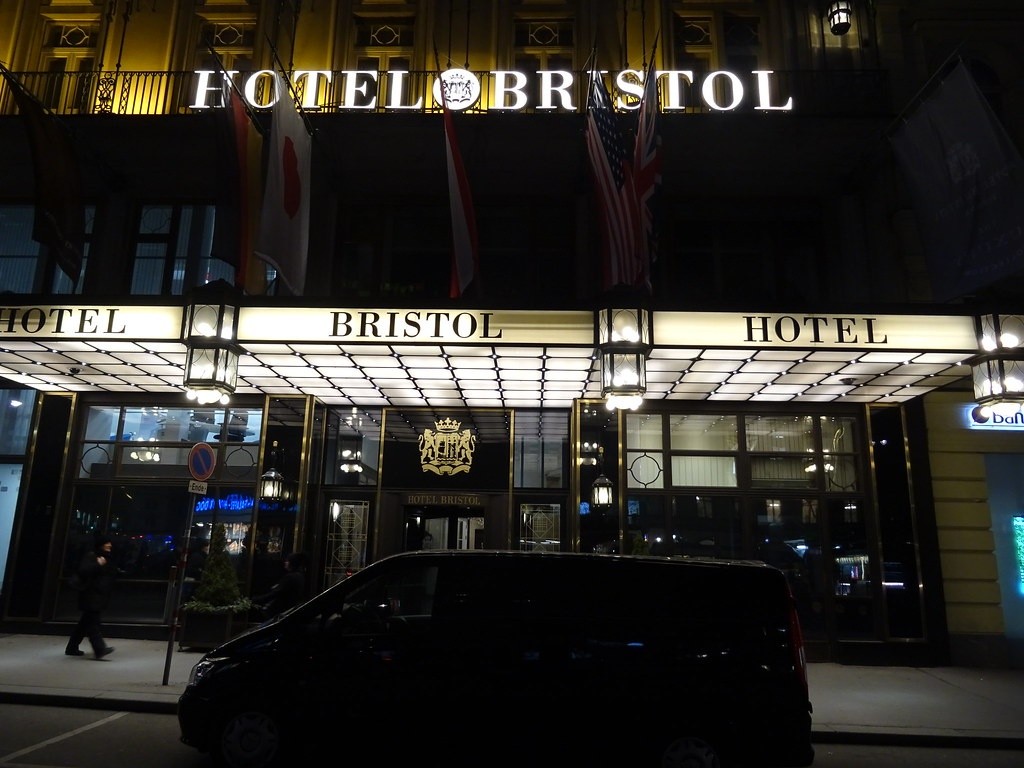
[94,536,111,547]
[288,551,306,567]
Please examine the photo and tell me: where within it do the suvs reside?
[175,547,815,768]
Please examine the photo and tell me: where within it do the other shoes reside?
[66,649,84,655]
[96,647,114,659]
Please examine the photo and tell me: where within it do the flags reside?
[2,70,88,284]
[581,65,652,294]
[229,89,267,294]
[442,100,479,298]
[256,64,311,297]
[632,56,666,263]
[887,55,1024,305]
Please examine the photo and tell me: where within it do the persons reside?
[262,553,307,618]
[186,540,209,577]
[65,536,115,658]
